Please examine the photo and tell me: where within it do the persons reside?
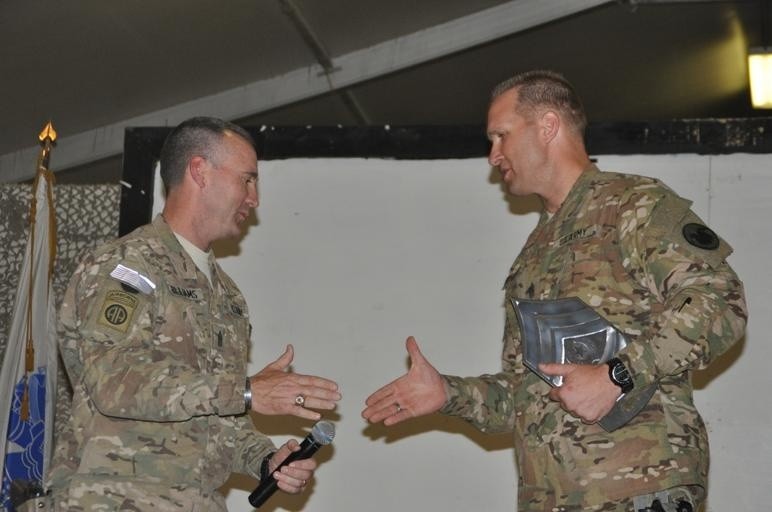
[51,116,345,512]
[356,66,752,512]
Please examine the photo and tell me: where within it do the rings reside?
[395,402,403,413]
[296,393,307,406]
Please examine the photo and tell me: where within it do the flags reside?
[0,164,62,512]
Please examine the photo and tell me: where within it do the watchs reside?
[607,359,632,394]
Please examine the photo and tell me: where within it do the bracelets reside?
[244,377,253,412]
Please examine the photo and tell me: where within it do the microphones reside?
[248,420,336,509]
